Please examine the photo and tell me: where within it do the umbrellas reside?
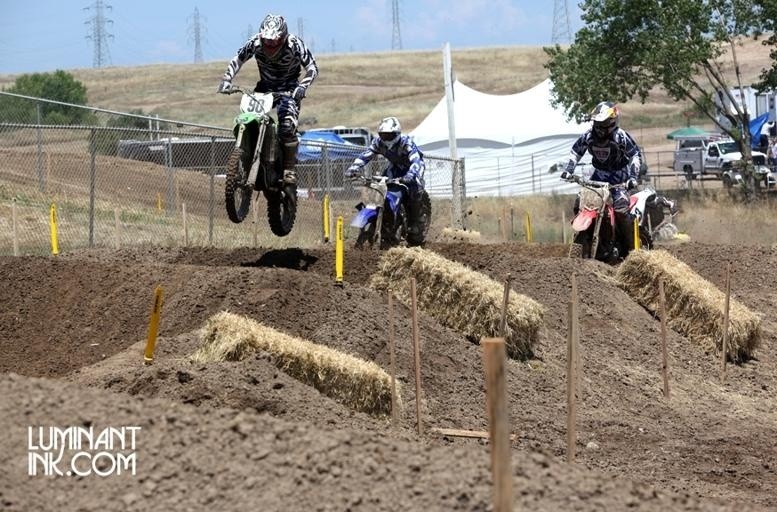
[666,127,709,142]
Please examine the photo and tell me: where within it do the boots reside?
[281,141,298,184]
[406,200,426,245]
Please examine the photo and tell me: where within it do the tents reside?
[410,76,598,196]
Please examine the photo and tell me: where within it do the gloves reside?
[217,80,232,94]
[293,85,306,101]
[560,167,573,179]
[625,175,638,191]
[345,163,360,178]
[402,172,415,184]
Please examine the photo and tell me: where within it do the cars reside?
[722,164,776,189]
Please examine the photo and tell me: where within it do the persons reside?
[345,116,426,235]
[760,120,775,154]
[219,13,319,184]
[770,139,777,166]
[561,101,642,252]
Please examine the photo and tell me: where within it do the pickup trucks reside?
[673,139,767,180]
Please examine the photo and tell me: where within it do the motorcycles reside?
[343,171,432,252]
[562,174,657,266]
[217,84,306,236]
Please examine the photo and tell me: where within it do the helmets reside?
[259,14,287,58]
[377,117,401,150]
[589,101,620,138]
